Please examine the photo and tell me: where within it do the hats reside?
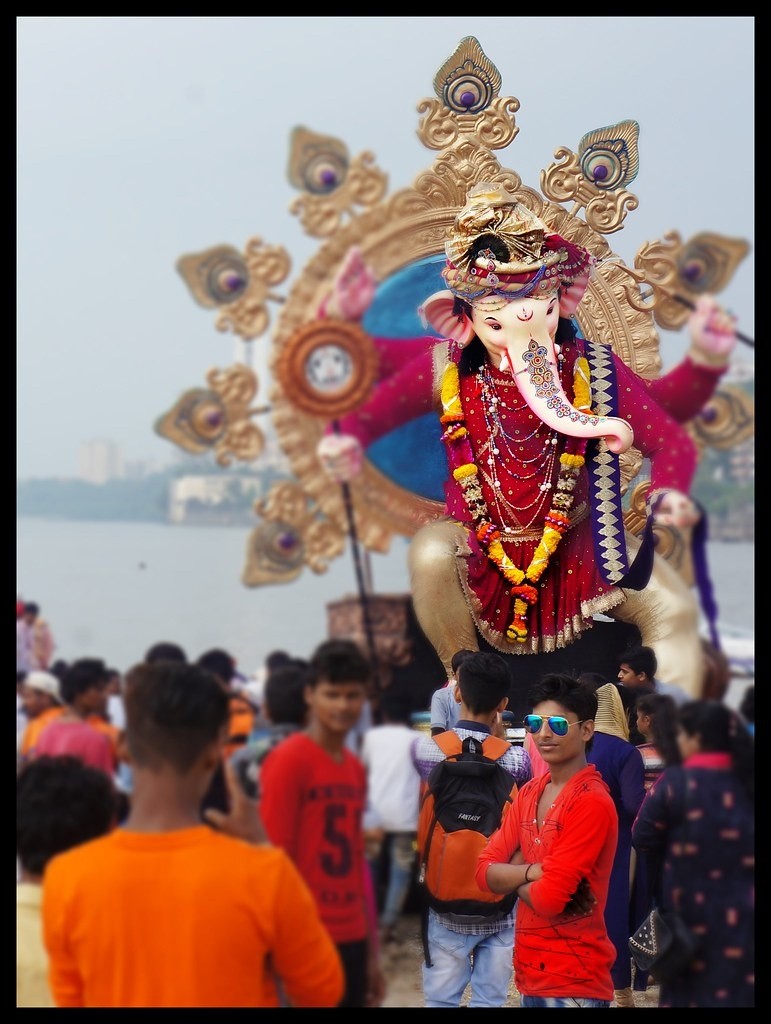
[24,670,65,709]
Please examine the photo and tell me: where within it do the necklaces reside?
[436,339,593,640]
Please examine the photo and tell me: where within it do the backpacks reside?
[411,727,525,970]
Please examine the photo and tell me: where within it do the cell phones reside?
[202,767,230,829]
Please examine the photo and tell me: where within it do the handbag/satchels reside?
[624,906,714,994]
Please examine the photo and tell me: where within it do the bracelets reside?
[525,863,533,881]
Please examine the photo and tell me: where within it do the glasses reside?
[522,713,588,738]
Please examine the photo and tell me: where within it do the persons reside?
[15,601,755,1007]
